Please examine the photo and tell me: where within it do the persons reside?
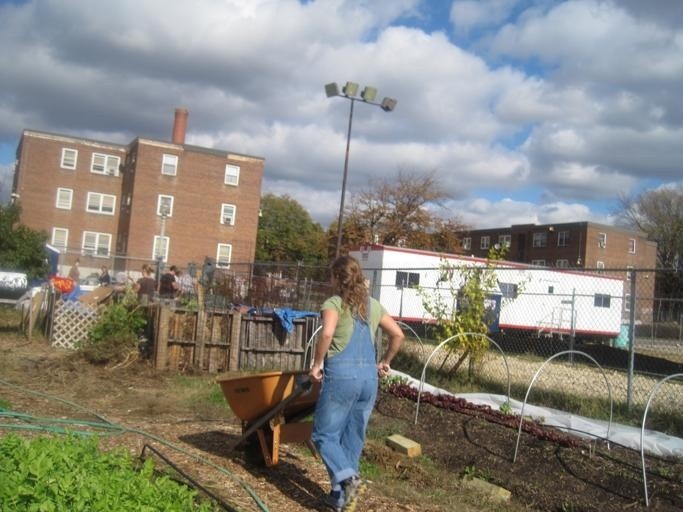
[66,257,194,304]
[308,255,407,511]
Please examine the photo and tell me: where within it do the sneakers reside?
[322,475,367,512]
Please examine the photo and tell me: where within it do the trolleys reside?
[215,368,321,467]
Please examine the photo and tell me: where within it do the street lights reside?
[323,82,396,263]
